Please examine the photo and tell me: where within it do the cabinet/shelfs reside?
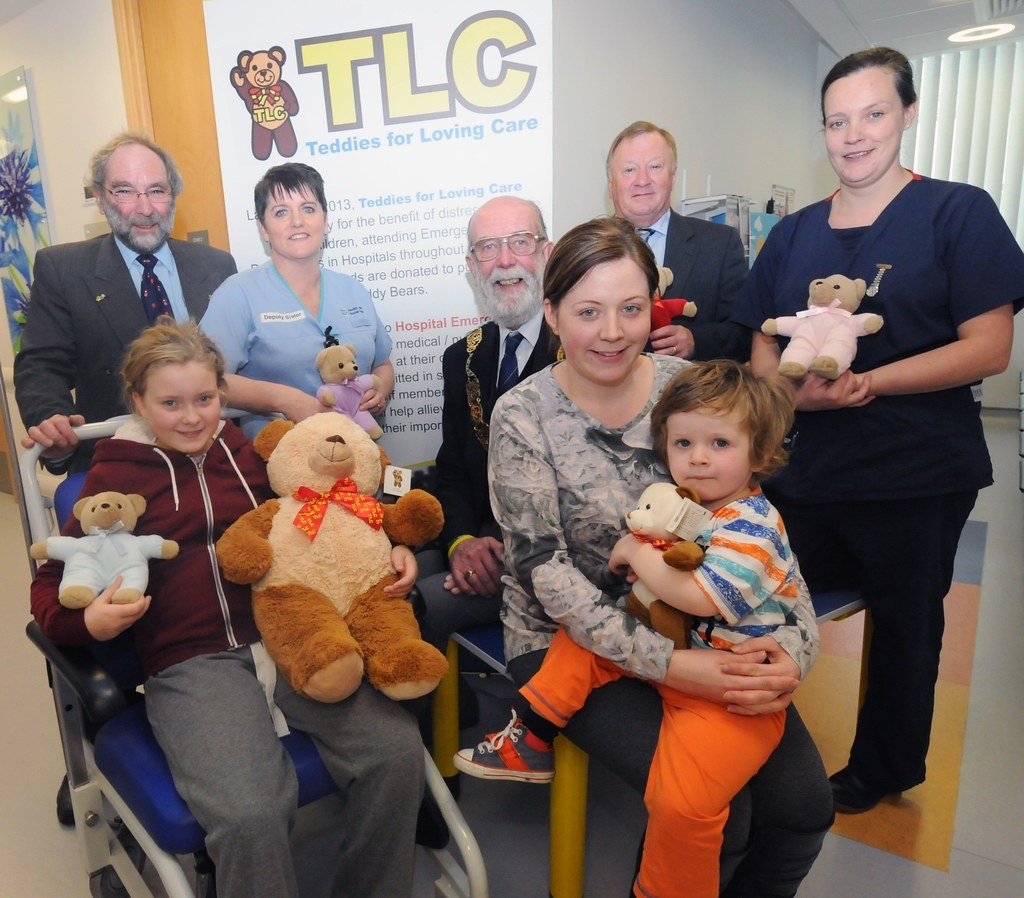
[683,197,755,259]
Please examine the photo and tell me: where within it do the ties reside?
[498,333,525,398]
[135,253,177,329]
[630,225,656,247]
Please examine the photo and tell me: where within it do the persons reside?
[198,163,393,442]
[14,134,239,475]
[454,358,799,897]
[28,315,422,898]
[409,199,556,746]
[487,217,833,897]
[744,45,1024,814]
[605,121,756,363]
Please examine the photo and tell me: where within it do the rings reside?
[674,347,676,353]
[378,405,382,408]
[465,570,475,579]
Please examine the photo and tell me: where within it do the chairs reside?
[19,407,488,898]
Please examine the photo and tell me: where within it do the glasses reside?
[91,180,173,204]
[470,231,547,261]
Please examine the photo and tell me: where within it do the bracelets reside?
[448,535,473,558]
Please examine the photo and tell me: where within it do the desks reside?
[430,590,868,898]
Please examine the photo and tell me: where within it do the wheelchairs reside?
[20,414,490,898]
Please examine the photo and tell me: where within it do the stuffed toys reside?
[650,262,697,352]
[29,490,180,608]
[623,481,700,649]
[761,274,884,379]
[316,345,384,441]
[215,412,450,700]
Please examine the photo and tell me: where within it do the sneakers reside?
[452,706,555,783]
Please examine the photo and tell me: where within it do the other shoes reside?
[828,763,885,814]
[416,778,462,849]
